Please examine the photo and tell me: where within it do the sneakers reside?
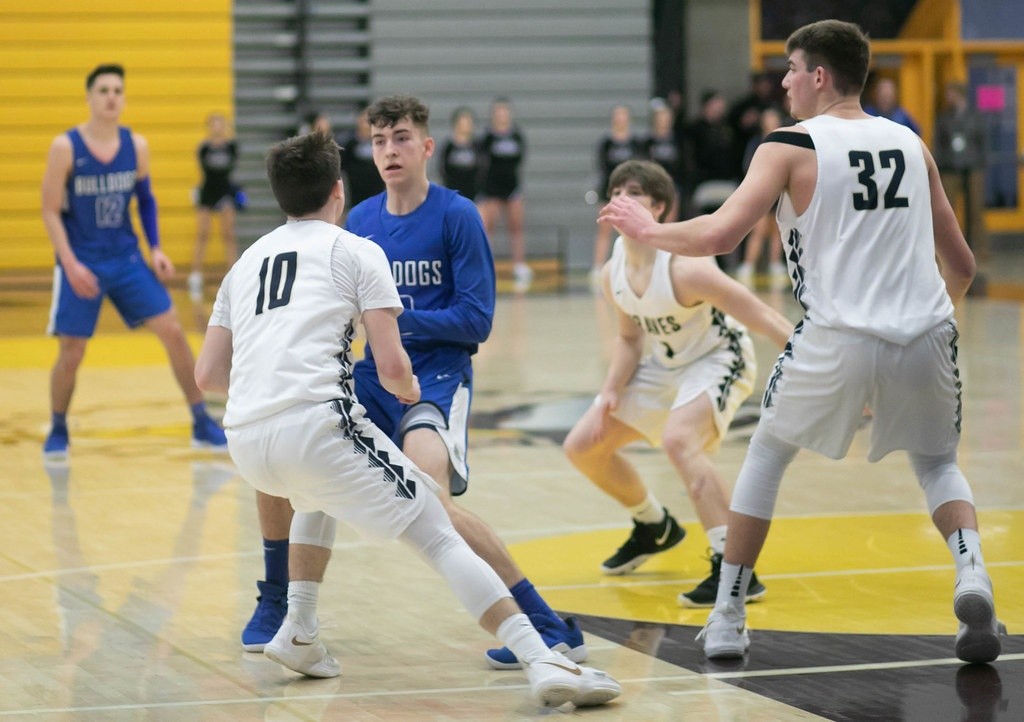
[953,569,1000,664]
[484,615,588,669]
[601,507,686,573]
[193,420,227,447]
[695,610,750,657]
[43,424,69,456]
[528,651,621,707]
[678,551,766,607]
[265,617,339,678]
[242,579,290,651]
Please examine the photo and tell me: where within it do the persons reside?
[436,97,532,295]
[597,73,989,301]
[598,20,1005,664]
[187,113,243,293]
[286,99,386,205]
[41,65,231,459]
[194,131,622,709]
[241,93,589,670]
[562,157,872,607]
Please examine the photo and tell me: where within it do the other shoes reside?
[512,263,534,282]
[769,262,791,288]
[188,271,203,289]
[738,263,755,288]
